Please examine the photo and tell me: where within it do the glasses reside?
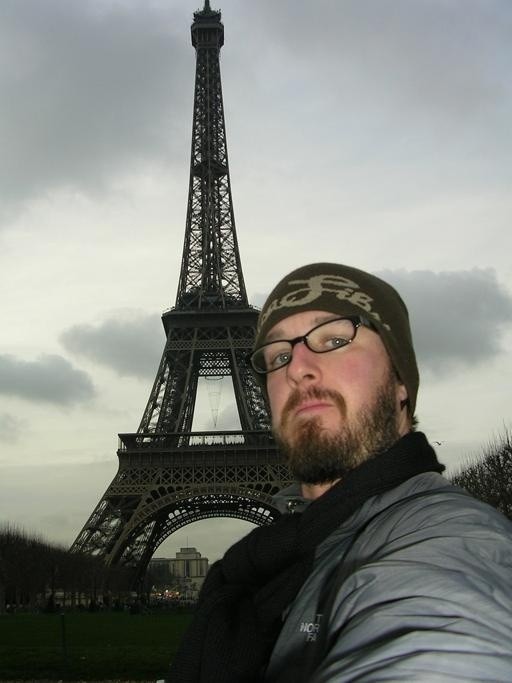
[245,318,361,375]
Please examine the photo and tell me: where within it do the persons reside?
[166,263,512,683]
[76,588,199,613]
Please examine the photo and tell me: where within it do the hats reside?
[253,263,418,416]
